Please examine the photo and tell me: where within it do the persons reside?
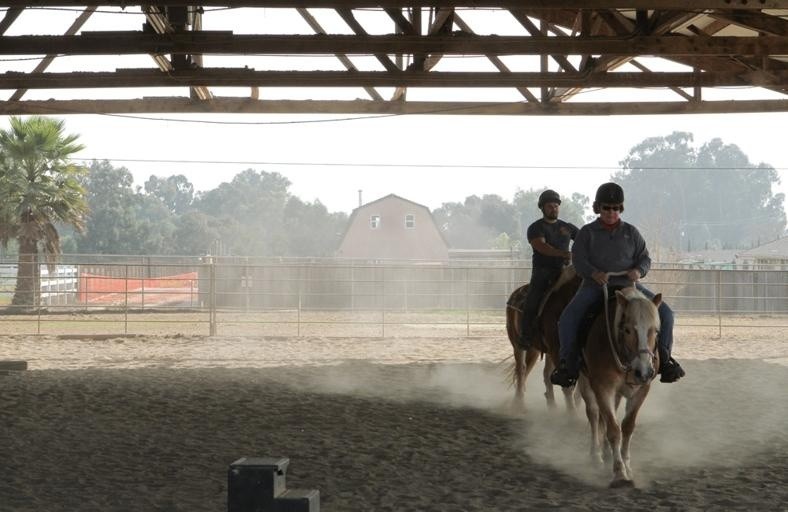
[518,188,580,348]
[550,182,686,387]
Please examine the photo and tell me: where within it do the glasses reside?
[599,203,619,211]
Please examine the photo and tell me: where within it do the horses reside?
[576,287,662,489]
[495,276,583,413]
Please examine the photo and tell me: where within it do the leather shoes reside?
[551,368,577,386]
[658,363,681,378]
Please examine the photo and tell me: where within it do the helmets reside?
[596,183,623,204]
[538,190,561,209]
